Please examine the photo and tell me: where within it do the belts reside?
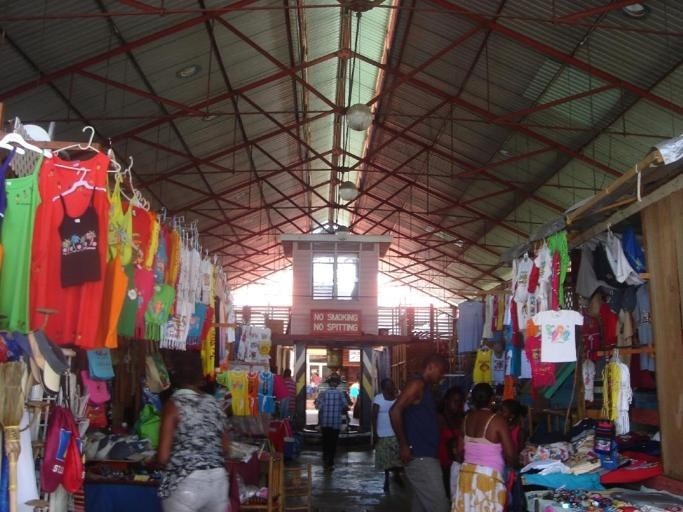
[196,465,217,470]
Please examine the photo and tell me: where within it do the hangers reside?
[475,337,519,355]
[510,230,570,265]
[600,345,630,378]
[0,116,231,283]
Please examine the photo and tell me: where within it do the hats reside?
[321,373,347,383]
[28,328,69,394]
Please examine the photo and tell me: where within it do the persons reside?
[315,373,349,470]
[156,371,231,512]
[371,354,528,512]
[283,368,297,416]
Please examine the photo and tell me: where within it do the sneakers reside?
[323,464,335,471]
[394,477,404,485]
[383,480,390,491]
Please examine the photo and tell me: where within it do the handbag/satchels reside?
[133,376,162,450]
[86,347,115,381]
[145,340,171,394]
[79,368,111,429]
[39,387,87,494]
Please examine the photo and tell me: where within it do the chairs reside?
[239,450,312,511]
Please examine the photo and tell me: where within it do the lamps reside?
[338,10,372,202]
